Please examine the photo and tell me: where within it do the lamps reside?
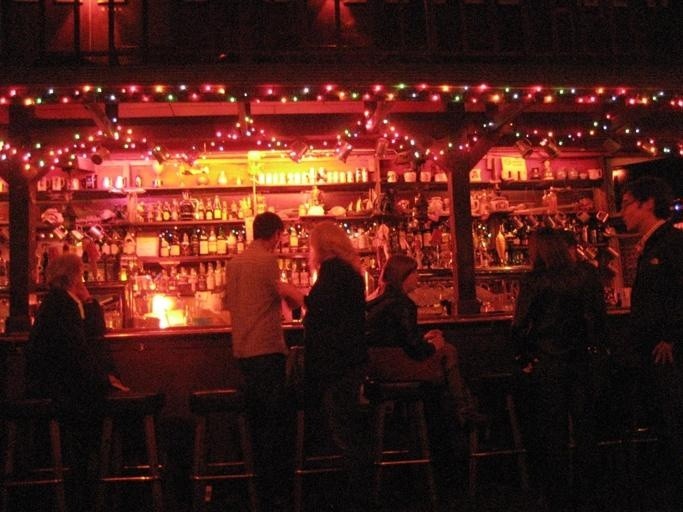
[373,137,390,159]
[514,138,534,160]
[336,140,353,165]
[289,139,308,162]
[150,143,172,167]
[87,135,108,167]
[543,141,562,159]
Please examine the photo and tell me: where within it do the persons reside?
[363,253,486,436]
[507,225,586,510]
[555,229,614,440]
[273,220,369,499]
[25,253,129,510]
[620,175,682,499]
[224,212,309,510]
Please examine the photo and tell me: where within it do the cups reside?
[51,223,104,240]
[589,167,602,179]
[35,174,127,190]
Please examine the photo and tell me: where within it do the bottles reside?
[274,222,377,283]
[556,167,588,179]
[253,167,375,216]
[377,216,447,249]
[132,161,248,187]
[137,190,253,295]
